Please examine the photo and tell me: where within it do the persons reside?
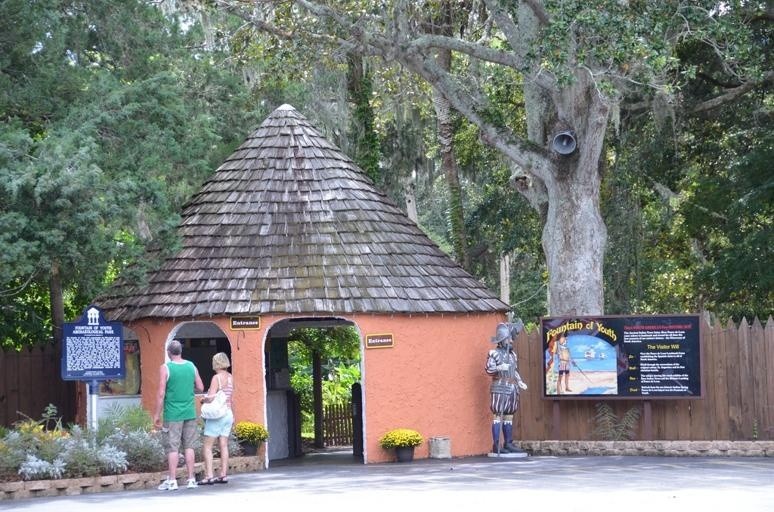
[483,321,529,455]
[152,339,206,491]
[554,336,577,395]
[195,351,235,486]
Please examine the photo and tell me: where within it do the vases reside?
[395,445,414,462]
[241,442,257,455]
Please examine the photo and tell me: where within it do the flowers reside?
[378,428,424,448]
[231,420,271,446]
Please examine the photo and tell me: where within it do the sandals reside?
[213,476,228,483]
[196,478,215,485]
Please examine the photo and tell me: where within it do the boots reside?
[557,375,562,394]
[565,373,572,392]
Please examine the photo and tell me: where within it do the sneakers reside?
[186,478,200,488]
[158,475,179,491]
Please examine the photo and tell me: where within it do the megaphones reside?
[552,130,577,155]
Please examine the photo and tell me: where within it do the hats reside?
[559,336,566,345]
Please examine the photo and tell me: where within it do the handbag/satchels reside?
[199,374,227,420]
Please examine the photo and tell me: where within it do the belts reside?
[560,359,569,362]
